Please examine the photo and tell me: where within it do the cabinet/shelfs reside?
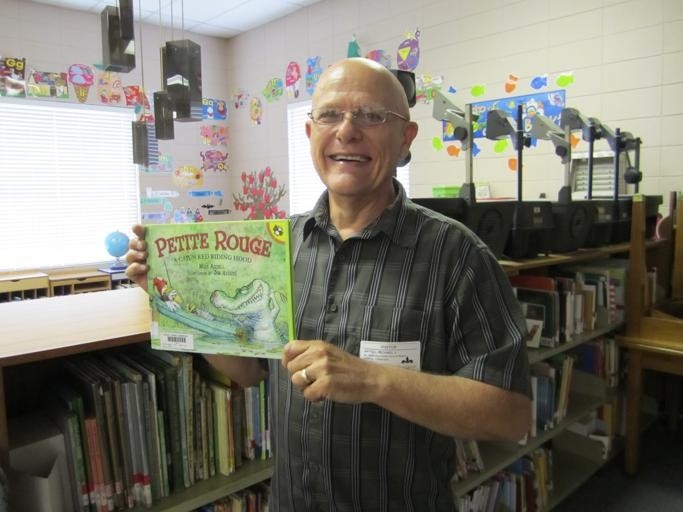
[0,189,683,512]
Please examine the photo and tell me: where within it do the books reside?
[142,219,297,360]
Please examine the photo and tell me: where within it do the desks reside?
[98,266,131,289]
[616,192,683,474]
[48,270,110,296]
[0,272,50,302]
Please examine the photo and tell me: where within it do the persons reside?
[124,56,537,511]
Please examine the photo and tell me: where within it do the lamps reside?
[96,0,203,166]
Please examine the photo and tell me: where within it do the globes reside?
[105,231,130,270]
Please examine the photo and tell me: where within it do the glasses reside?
[307,102,408,125]
